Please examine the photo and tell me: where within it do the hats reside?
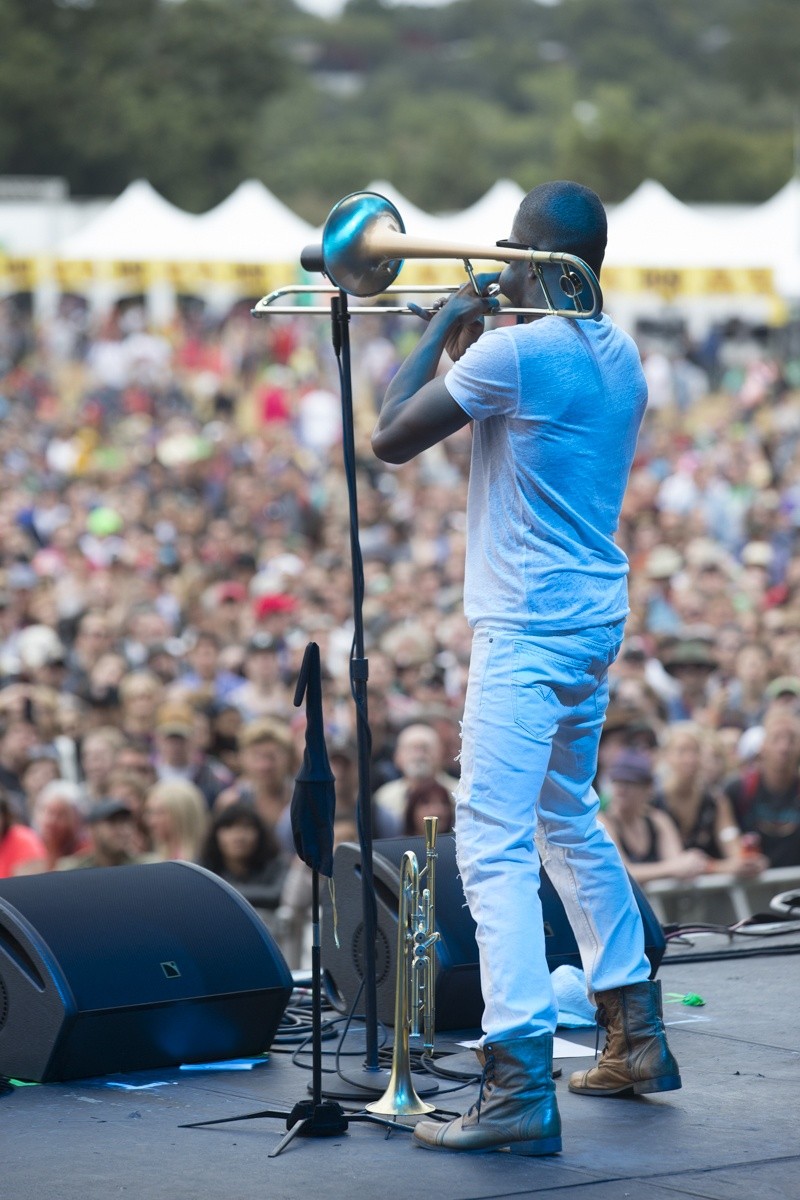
[232,717,298,750]
[152,704,195,741]
[604,701,662,742]
[658,642,719,672]
[763,676,800,702]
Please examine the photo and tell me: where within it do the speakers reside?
[318,832,667,1033]
[1,860,295,1084]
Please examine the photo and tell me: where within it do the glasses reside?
[493,238,543,266]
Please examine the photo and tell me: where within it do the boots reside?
[411,1034,565,1157]
[567,979,685,1097]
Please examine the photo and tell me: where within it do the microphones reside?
[300,245,326,273]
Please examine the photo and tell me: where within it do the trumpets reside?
[367,814,441,1118]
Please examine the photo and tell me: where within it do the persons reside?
[366,182,680,1160]
[357,302,800,884]
[0,295,357,967]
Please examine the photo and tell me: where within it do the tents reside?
[1,175,797,314]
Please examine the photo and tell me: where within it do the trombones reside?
[250,191,605,320]
